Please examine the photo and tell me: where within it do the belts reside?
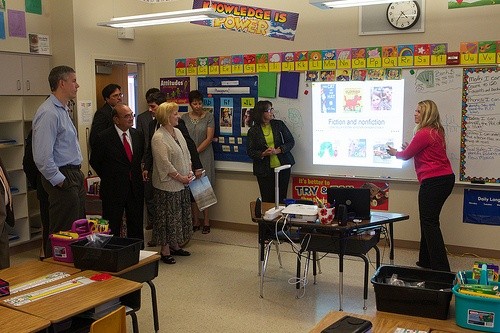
[61,164,81,169]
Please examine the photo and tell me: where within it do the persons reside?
[246,100,295,244]
[31,66,85,259]
[385,100,455,272]
[151,102,194,264]
[88,83,124,220]
[88,103,145,250]
[242,108,254,127]
[220,108,232,127]
[180,90,216,234]
[134,88,204,248]
[22,94,50,259]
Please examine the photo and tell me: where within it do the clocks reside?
[386,0,421,30]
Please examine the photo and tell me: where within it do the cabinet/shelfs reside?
[0,51,52,247]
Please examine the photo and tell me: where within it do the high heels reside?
[202,226,210,234]
[194,225,200,232]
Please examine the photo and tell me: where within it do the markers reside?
[337,174,348,176]
[353,175,367,177]
[379,176,391,178]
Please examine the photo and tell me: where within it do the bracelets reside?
[174,172,179,178]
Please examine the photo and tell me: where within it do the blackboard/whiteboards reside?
[196,64,499,187]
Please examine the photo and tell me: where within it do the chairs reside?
[89,306,127,333]
[258,220,322,298]
[296,227,382,310]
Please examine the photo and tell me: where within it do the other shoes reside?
[160,253,176,264]
[40,256,45,260]
[170,248,191,256]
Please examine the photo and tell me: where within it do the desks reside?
[0,245,162,333]
[307,310,430,333]
[252,206,409,312]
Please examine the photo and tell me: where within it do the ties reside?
[0,166,12,212]
[122,133,133,160]
[156,123,161,130]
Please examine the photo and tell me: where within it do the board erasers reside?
[471,180,485,184]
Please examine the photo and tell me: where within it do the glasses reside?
[264,108,274,113]
[120,112,134,119]
[113,93,123,99]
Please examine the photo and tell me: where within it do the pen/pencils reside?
[316,196,336,208]
[456,270,468,285]
[299,220,314,223]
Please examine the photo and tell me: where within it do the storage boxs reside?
[451,271,500,333]
[70,235,143,273]
[49,218,112,263]
[370,262,457,320]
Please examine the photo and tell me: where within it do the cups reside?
[318,207,335,224]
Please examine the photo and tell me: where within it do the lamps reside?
[320,0,412,9]
[98,0,228,30]
